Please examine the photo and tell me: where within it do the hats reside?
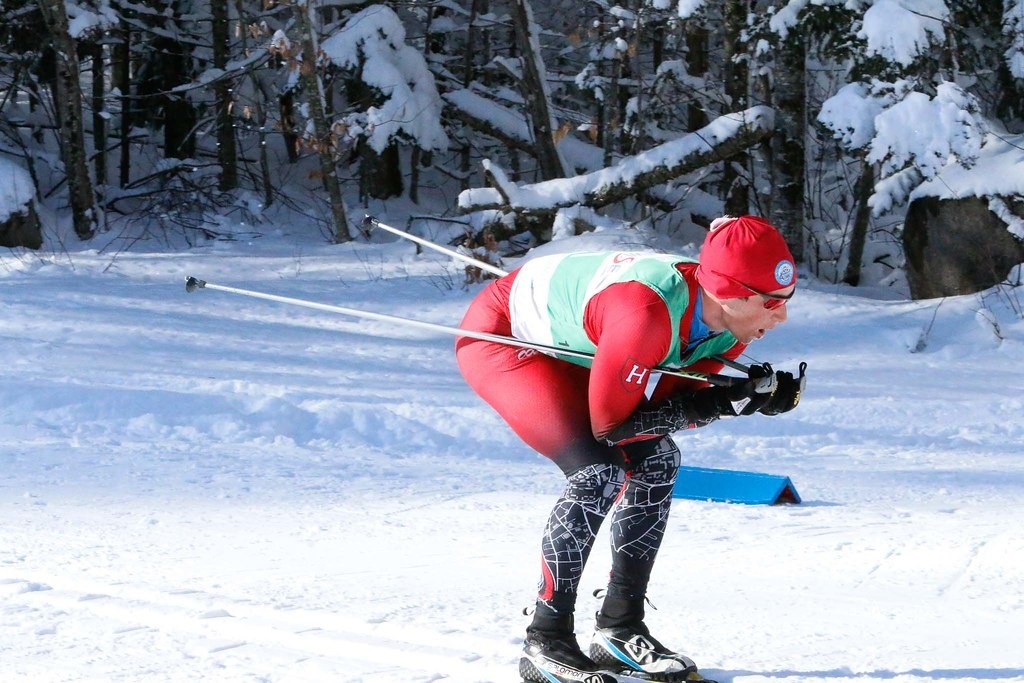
[696,216,795,298]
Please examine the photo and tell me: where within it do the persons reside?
[454,215,807,683]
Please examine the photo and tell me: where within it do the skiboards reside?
[521,659,720,683]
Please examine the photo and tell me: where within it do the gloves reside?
[758,363,808,417]
[696,363,777,421]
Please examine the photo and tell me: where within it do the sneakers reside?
[589,610,697,683]
[519,625,624,683]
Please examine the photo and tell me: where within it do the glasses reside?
[711,271,795,311]
